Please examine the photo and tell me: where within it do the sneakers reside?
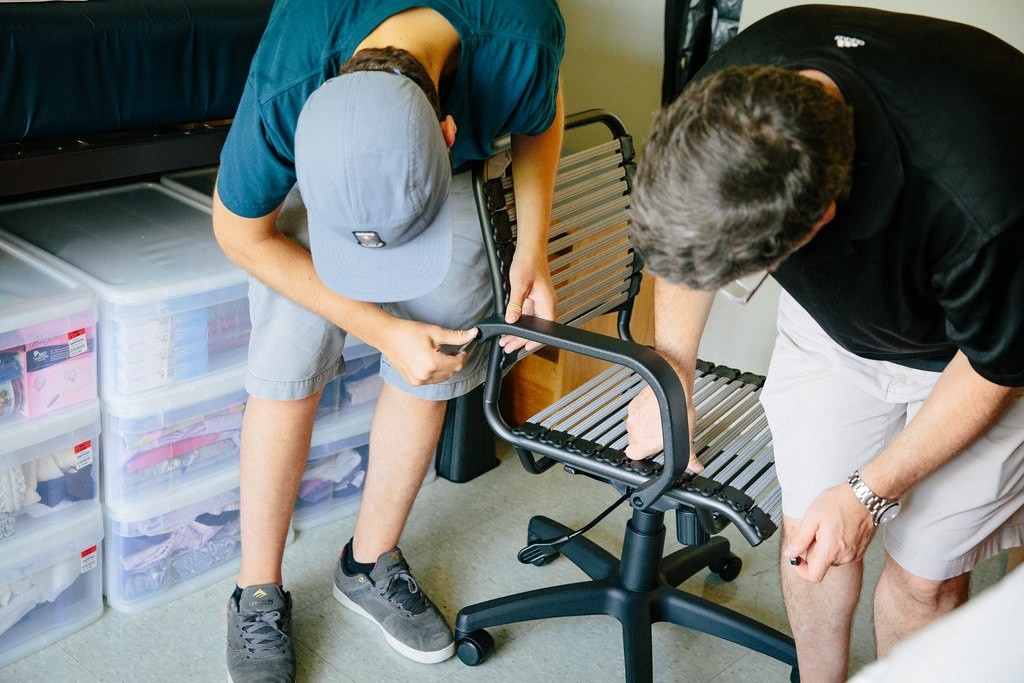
[332,542,456,664]
[226,583,296,683]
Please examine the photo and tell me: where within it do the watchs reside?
[847,470,901,526]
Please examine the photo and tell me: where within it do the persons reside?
[211,0,565,682]
[626,4,1024,682]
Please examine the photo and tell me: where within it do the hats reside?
[294,69,455,303]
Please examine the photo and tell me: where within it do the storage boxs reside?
[0,164,380,665]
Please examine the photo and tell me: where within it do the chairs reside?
[459,113,796,683]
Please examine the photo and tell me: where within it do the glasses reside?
[718,267,769,304]
